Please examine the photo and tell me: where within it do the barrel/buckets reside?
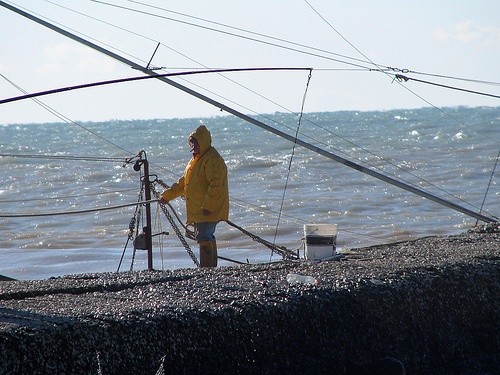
[302,223,338,260]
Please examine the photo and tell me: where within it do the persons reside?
[158,125,229,269]
